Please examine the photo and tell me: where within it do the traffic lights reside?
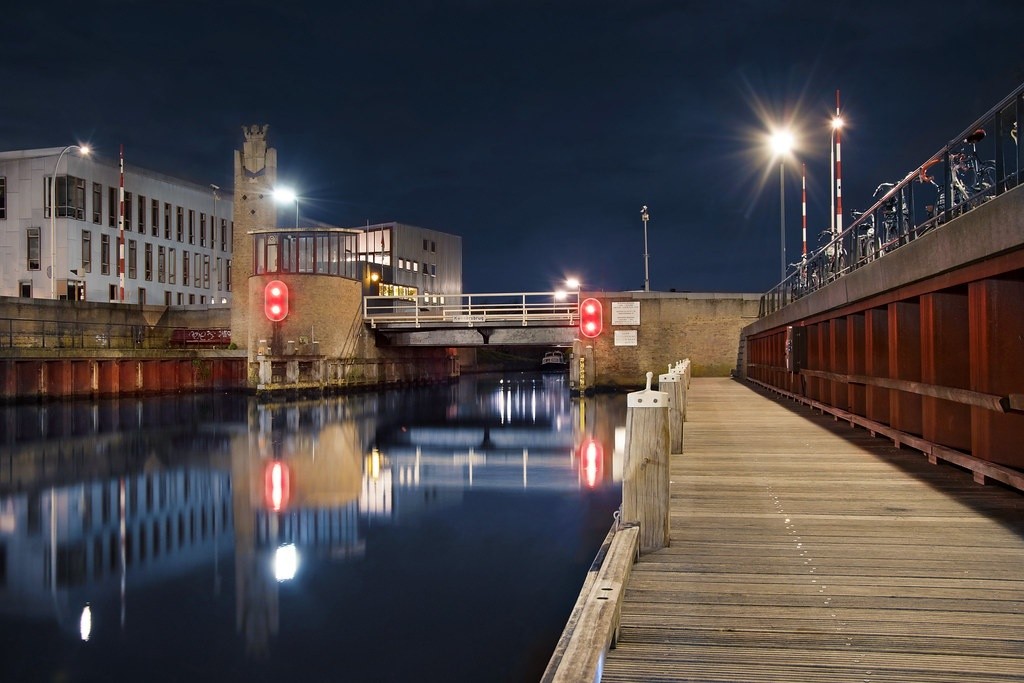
[580,297,603,338]
[264,280,289,322]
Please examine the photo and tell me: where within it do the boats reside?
[540,350,569,373]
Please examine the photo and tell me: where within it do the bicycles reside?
[784,119,1018,303]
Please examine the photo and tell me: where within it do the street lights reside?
[830,117,845,247]
[50,144,89,297]
[771,129,794,282]
[277,190,299,227]
[568,279,580,315]
